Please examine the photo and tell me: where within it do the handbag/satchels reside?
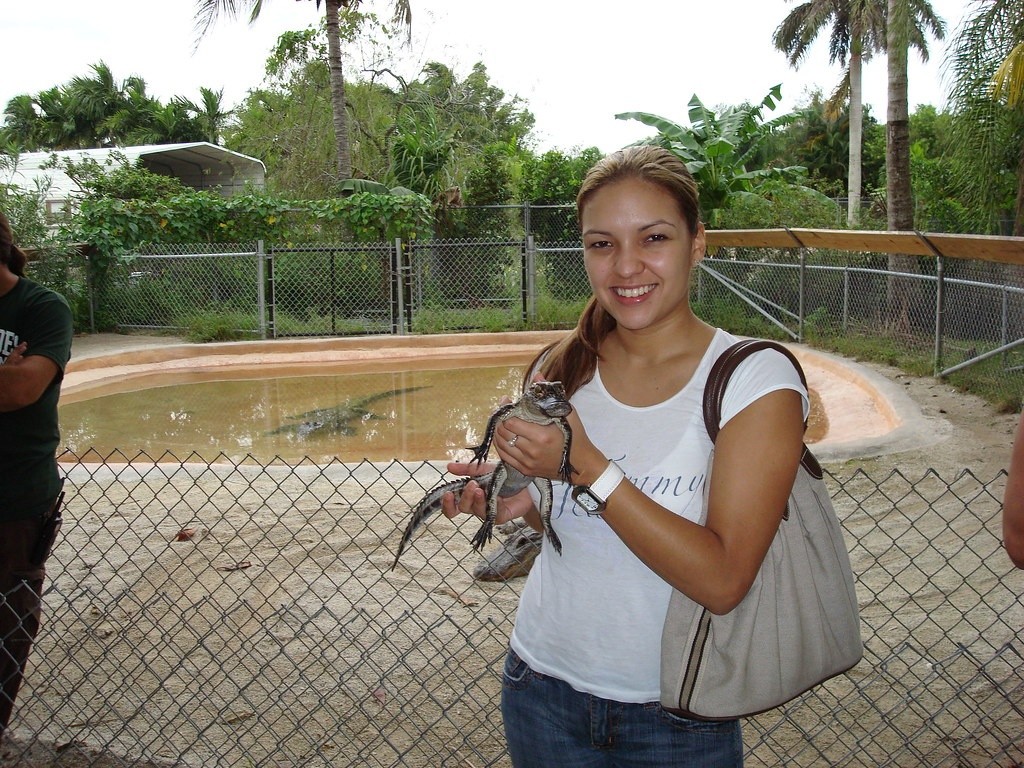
[660,339,863,722]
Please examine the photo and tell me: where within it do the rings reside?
[509,434,518,447]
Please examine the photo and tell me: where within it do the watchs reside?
[571,459,626,515]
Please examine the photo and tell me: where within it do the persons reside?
[441,144,811,768]
[1002,406,1024,569]
[0,210,73,734]
